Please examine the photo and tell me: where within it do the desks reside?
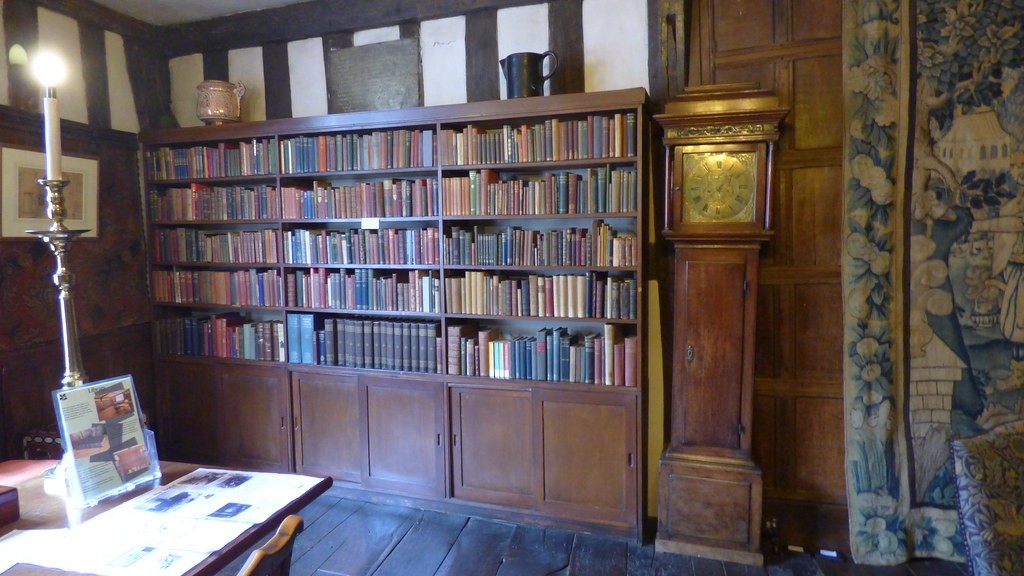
[0,459,333,576]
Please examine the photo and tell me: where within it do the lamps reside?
[24,53,92,390]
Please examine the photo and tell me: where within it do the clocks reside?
[652,81,792,568]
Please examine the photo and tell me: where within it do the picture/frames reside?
[0,142,103,243]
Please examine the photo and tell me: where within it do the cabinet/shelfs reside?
[137,85,657,548]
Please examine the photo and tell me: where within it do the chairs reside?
[236,513,305,576]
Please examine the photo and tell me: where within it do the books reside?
[143,111,637,388]
